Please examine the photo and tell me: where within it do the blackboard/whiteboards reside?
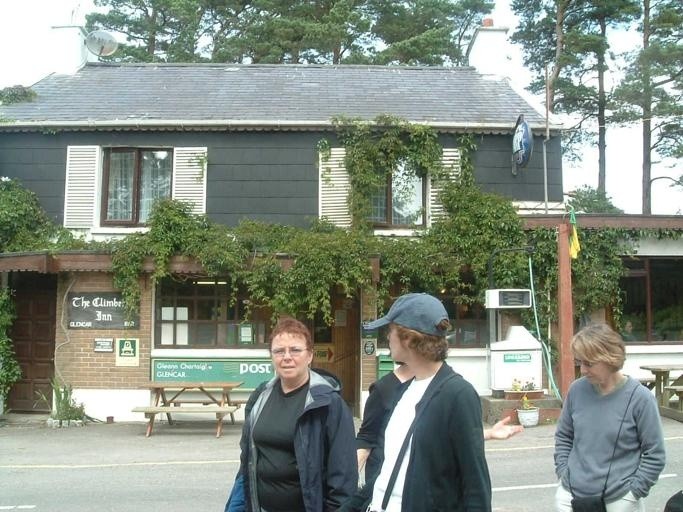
[67,292,140,330]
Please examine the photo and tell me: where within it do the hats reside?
[360,290,449,337]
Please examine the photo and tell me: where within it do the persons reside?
[339,290,492,512]
[224,314,358,511]
[354,361,416,491]
[550,323,665,511]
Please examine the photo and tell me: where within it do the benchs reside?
[638,376,679,392]
[131,405,237,419]
[664,385,683,411]
[160,398,248,409]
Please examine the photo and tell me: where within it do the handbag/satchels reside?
[570,495,607,512]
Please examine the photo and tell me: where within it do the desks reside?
[139,382,245,439]
[640,363,683,408]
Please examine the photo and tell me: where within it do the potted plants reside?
[516,396,540,427]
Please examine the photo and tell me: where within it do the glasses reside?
[268,347,309,358]
[571,357,598,368]
